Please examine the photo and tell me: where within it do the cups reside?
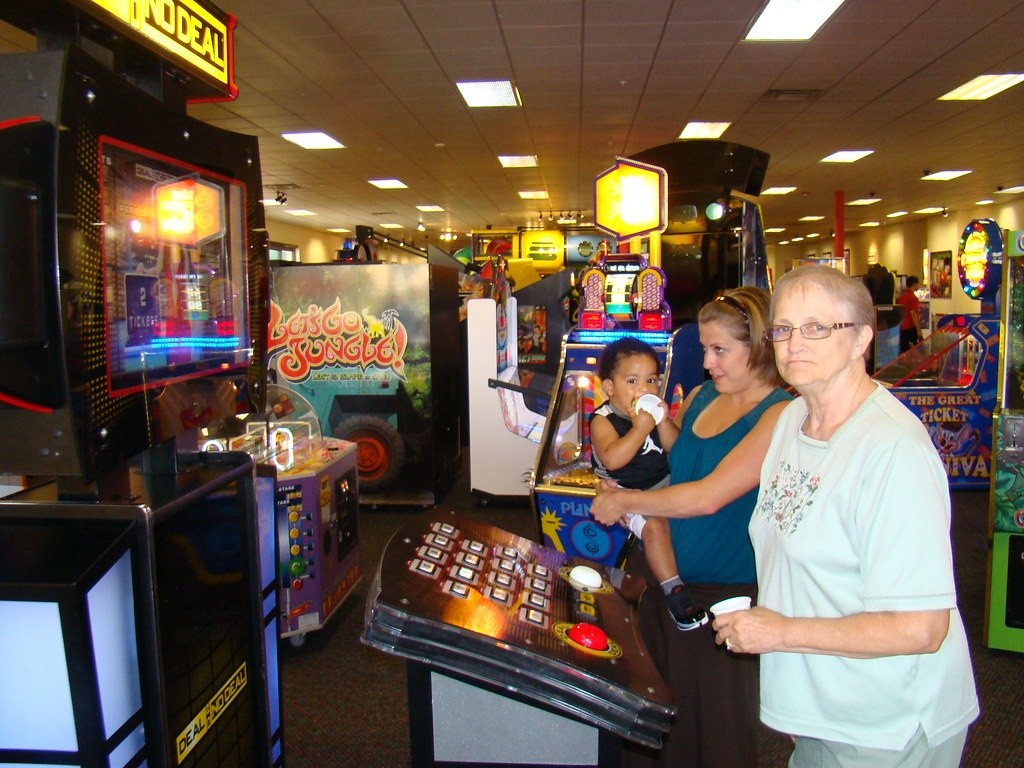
[710,596,752,648]
[631,394,664,425]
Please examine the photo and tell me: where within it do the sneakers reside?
[663,584,710,631]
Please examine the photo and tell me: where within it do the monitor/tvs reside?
[98,134,251,397]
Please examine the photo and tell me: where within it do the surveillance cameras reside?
[870,193,876,197]
[997,186,1004,191]
[924,171,929,176]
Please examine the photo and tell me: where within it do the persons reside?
[588,286,798,768]
[712,264,980,768]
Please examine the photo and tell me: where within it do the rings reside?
[725,638,735,650]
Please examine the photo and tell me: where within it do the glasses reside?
[763,322,855,342]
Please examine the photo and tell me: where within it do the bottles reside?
[343,243,352,262]
[930,259,951,297]
